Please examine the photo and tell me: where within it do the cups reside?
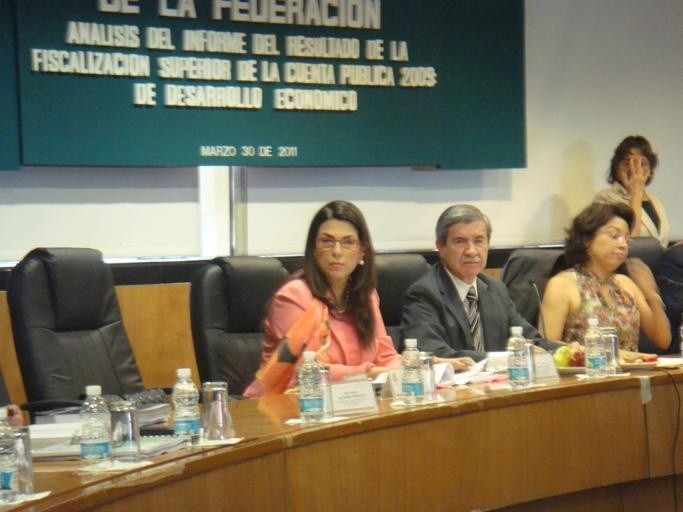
[600,328,622,374]
[201,381,235,446]
[420,352,437,404]
[524,343,536,388]
[13,426,34,504]
[111,401,144,470]
[314,366,334,422]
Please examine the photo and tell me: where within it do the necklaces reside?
[323,272,353,314]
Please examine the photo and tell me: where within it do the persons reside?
[538,200,672,353]
[592,136,683,251]
[396,204,587,369]
[242,199,478,398]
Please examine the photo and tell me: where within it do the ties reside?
[468,286,484,351]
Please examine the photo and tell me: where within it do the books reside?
[28,434,190,462]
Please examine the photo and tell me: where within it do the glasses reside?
[315,235,360,256]
[595,231,635,247]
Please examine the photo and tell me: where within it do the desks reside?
[0,367,683,512]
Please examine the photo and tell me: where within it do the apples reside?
[553,346,585,366]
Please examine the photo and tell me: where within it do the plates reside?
[620,363,659,370]
[556,366,586,375]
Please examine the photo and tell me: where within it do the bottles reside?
[507,327,526,390]
[584,316,602,377]
[172,368,203,455]
[299,351,321,417]
[79,386,111,471]
[1,406,19,504]
[401,339,421,404]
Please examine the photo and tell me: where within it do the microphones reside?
[658,276,683,288]
[528,278,548,350]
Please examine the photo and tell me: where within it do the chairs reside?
[5,247,203,424]
[190,254,292,401]
[621,236,662,276]
[507,250,568,326]
[376,253,430,353]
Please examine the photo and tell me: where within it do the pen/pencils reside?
[8,405,14,416]
[462,360,473,364]
